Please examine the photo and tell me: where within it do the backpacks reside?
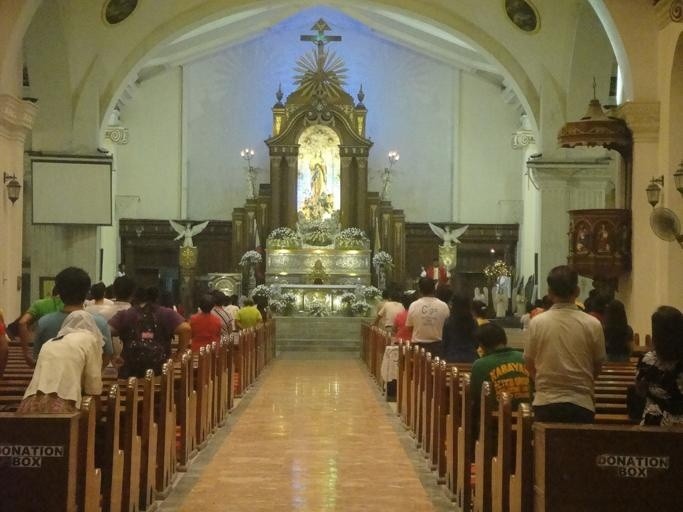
[122,302,169,375]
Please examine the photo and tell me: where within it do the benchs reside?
[355,316,682,512]
[0,308,277,512]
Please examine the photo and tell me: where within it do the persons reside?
[310,150,327,197]
[312,40,329,69]
[444,226,462,246]
[634,306,683,426]
[173,222,194,247]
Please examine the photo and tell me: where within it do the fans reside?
[648,207,682,250]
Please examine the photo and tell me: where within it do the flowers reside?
[266,223,369,251]
[240,280,384,318]
[480,259,511,283]
[371,249,394,270]
[236,251,264,268]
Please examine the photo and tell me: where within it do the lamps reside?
[2,171,22,208]
[645,173,664,207]
[238,145,260,202]
[378,148,400,201]
[673,163,682,197]
